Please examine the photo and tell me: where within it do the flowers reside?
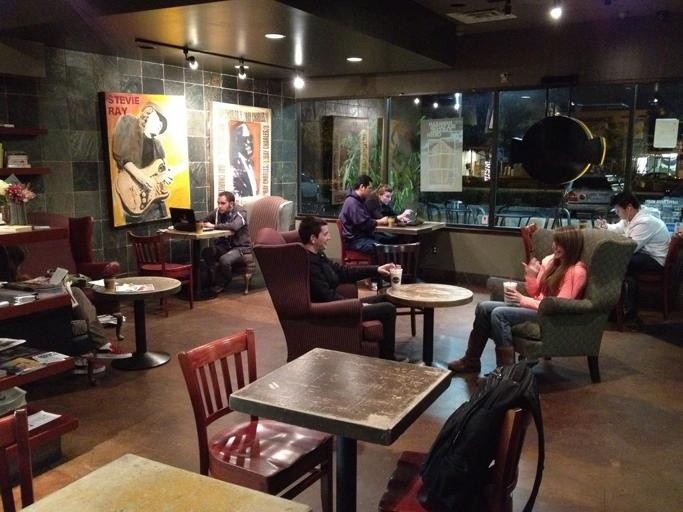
[0,179,37,205]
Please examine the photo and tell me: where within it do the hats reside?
[397,209,416,223]
[142,102,167,134]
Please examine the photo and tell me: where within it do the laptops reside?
[170,205,204,234]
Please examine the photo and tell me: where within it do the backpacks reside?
[418,359,545,512]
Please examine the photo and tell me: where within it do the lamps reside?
[134,35,307,79]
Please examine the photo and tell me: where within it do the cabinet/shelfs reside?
[0,124,50,175]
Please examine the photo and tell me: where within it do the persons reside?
[449,227,587,372]
[299,215,408,363]
[595,191,671,332]
[197,191,252,299]
[338,176,390,290]
[365,183,415,244]
[230,122,258,197]
[112,101,174,224]
[0,245,133,374]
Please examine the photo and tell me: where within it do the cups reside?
[195,220,203,234]
[103,273,117,292]
[503,281,518,305]
[390,268,403,288]
[387,215,396,227]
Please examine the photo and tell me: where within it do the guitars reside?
[115,158,189,217]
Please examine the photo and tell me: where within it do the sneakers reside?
[95,342,133,359]
[387,353,410,363]
[74,359,106,374]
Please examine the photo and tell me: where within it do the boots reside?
[496,347,515,366]
[448,335,488,373]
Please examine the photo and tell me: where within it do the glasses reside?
[242,135,253,142]
